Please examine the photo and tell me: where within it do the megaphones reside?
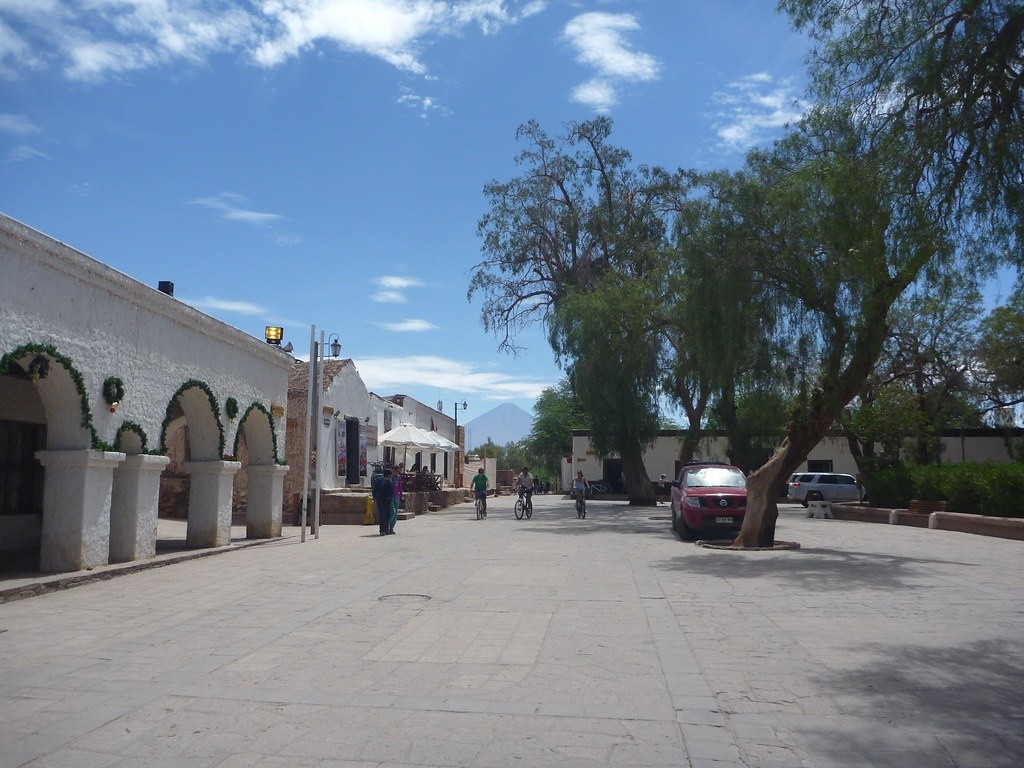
[282,341,295,354]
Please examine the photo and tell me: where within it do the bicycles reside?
[513,487,533,519]
[470,489,489,520]
[575,487,590,520]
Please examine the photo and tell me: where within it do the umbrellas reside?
[408,429,462,472]
[378,423,436,474]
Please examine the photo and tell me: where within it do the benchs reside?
[807,500,833,519]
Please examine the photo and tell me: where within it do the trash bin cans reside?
[299,497,311,517]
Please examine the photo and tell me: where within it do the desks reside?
[424,475,441,490]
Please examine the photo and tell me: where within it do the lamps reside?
[266,326,284,348]
[364,416,370,422]
[334,410,340,417]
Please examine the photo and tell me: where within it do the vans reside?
[787,472,867,508]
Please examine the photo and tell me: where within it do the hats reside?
[576,470,582,475]
[521,466,528,470]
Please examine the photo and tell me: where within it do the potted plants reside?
[910,469,960,516]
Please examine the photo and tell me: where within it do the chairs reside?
[399,474,442,492]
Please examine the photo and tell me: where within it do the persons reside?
[658,474,666,504]
[533,475,550,495]
[621,472,626,493]
[470,468,490,517]
[371,466,404,535]
[514,466,535,515]
[399,462,431,480]
[573,470,590,512]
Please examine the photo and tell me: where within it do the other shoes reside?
[527,510,530,514]
[483,512,487,516]
[384,530,391,535]
[380,530,386,536]
[390,530,396,534]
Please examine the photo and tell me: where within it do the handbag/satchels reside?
[372,478,383,499]
[656,486,665,495]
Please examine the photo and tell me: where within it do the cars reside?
[671,464,749,539]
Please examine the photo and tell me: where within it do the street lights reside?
[454,400,467,433]
[315,329,342,540]
